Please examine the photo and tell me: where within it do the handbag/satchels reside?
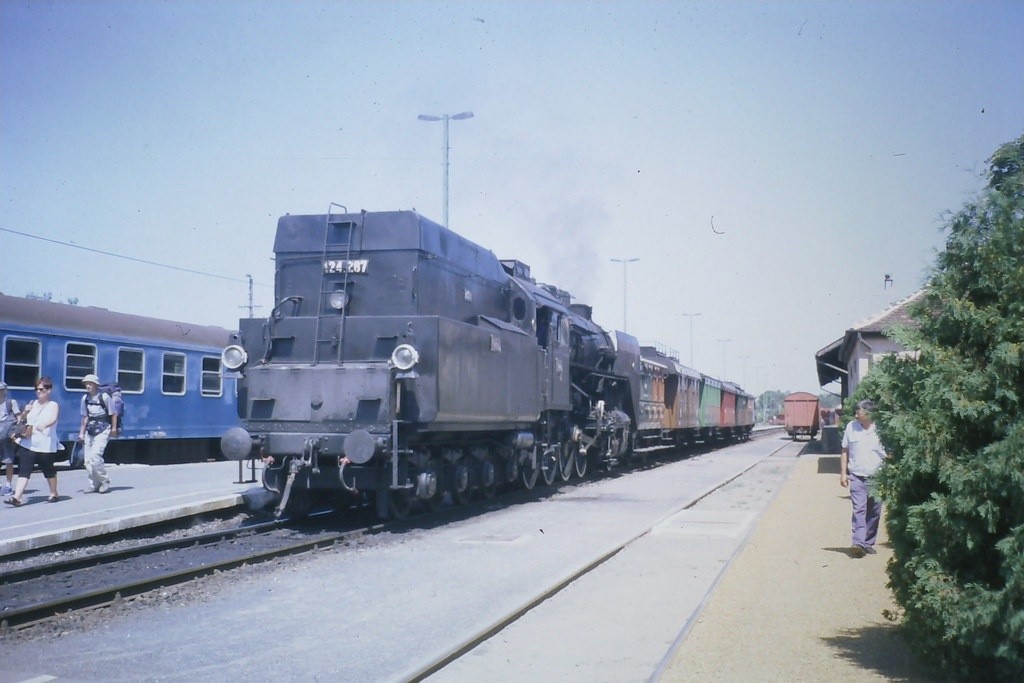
[0,399,23,440]
[8,400,35,444]
[85,422,109,436]
[70,439,84,468]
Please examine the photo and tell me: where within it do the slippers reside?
[3,497,21,507]
[47,496,59,501]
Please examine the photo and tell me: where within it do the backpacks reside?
[85,383,125,433]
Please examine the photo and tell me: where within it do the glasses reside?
[34,387,48,391]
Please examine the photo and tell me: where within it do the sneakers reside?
[1,487,15,496]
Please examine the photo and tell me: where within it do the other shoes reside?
[99,483,109,492]
[852,544,863,557]
[83,487,99,493]
[865,547,876,554]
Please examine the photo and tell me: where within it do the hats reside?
[82,375,100,385]
[0,382,7,389]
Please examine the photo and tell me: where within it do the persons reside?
[3,376,59,506]
[79,374,117,494]
[840,398,887,558]
[0,382,20,495]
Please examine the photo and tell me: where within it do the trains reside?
[0,293,236,455]
[783,392,819,441]
[218,202,756,527]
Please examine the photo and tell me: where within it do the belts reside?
[851,473,874,483]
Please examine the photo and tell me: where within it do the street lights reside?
[416,111,477,230]
[608,258,642,334]
[681,312,702,371]
[717,339,731,382]
